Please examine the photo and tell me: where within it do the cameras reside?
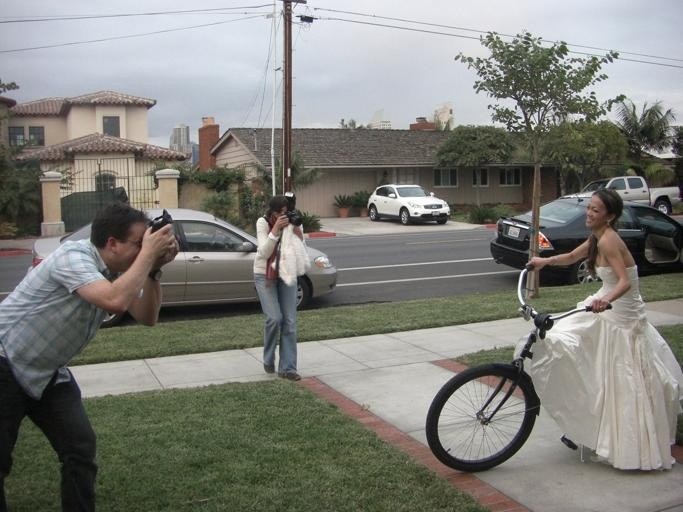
[285,191,302,226]
[149,209,186,248]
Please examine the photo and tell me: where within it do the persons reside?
[253,195,308,381]
[513,187,683,472]
[0,202,181,511]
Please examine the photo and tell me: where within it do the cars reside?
[490,196,683,284]
[26,208,337,328]
[367,184,450,225]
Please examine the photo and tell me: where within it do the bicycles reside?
[425,265,612,474]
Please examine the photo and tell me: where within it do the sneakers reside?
[263,364,301,382]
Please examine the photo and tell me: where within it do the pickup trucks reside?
[562,176,681,217]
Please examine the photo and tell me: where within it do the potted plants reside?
[333,189,372,217]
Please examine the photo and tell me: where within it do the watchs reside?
[148,269,163,281]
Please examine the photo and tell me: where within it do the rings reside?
[600,305,605,309]
